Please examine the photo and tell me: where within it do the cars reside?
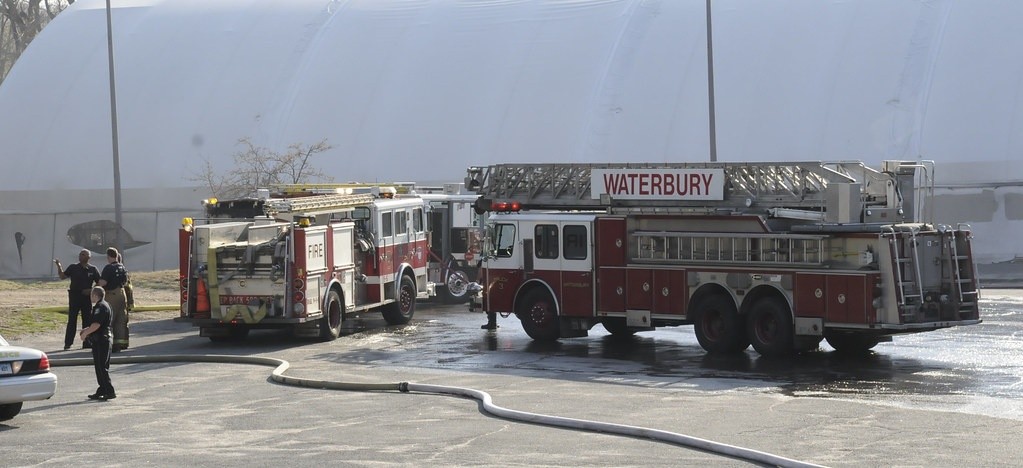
[0,335,57,422]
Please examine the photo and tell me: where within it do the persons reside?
[52,249,100,350]
[97,246,134,353]
[79,286,116,401]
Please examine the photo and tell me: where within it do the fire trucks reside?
[461,159,983,364]
[173,182,492,342]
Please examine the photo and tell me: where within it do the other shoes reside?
[87,392,105,399]
[102,395,115,400]
[111,343,127,353]
[82,343,92,348]
[481,325,497,329]
[63,345,74,350]
